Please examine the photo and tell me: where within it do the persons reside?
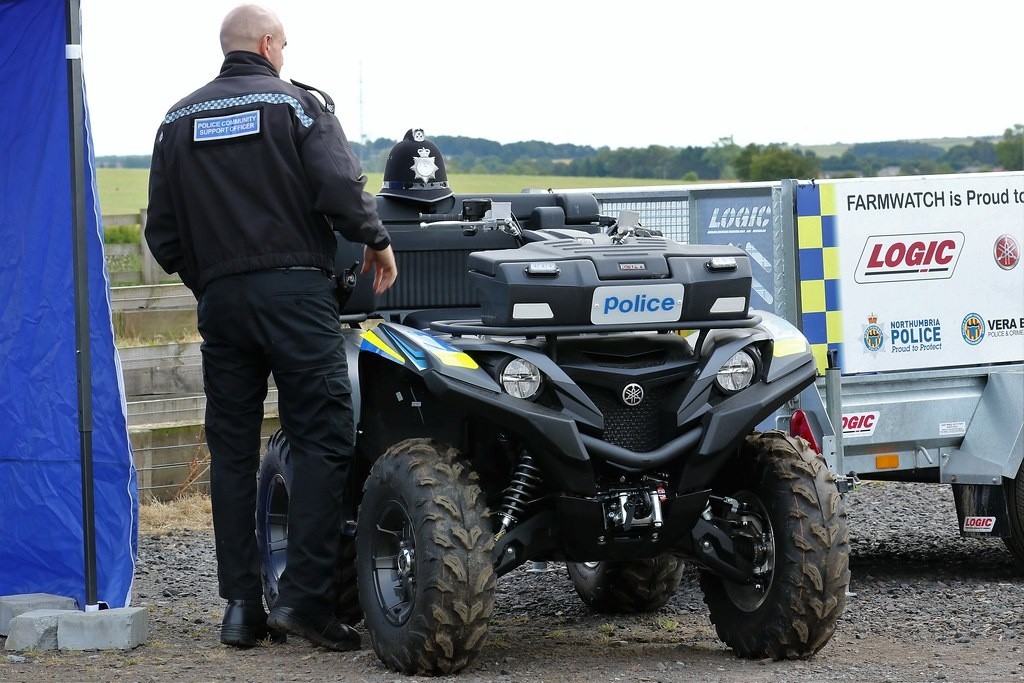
[144,5,398,651]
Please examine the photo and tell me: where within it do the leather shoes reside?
[266,596,361,651]
[219,603,285,649]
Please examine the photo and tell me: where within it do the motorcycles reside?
[258,192,852,674]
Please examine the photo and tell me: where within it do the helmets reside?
[374,130,452,203]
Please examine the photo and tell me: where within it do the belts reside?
[276,266,323,271]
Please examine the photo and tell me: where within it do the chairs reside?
[384,224,525,332]
